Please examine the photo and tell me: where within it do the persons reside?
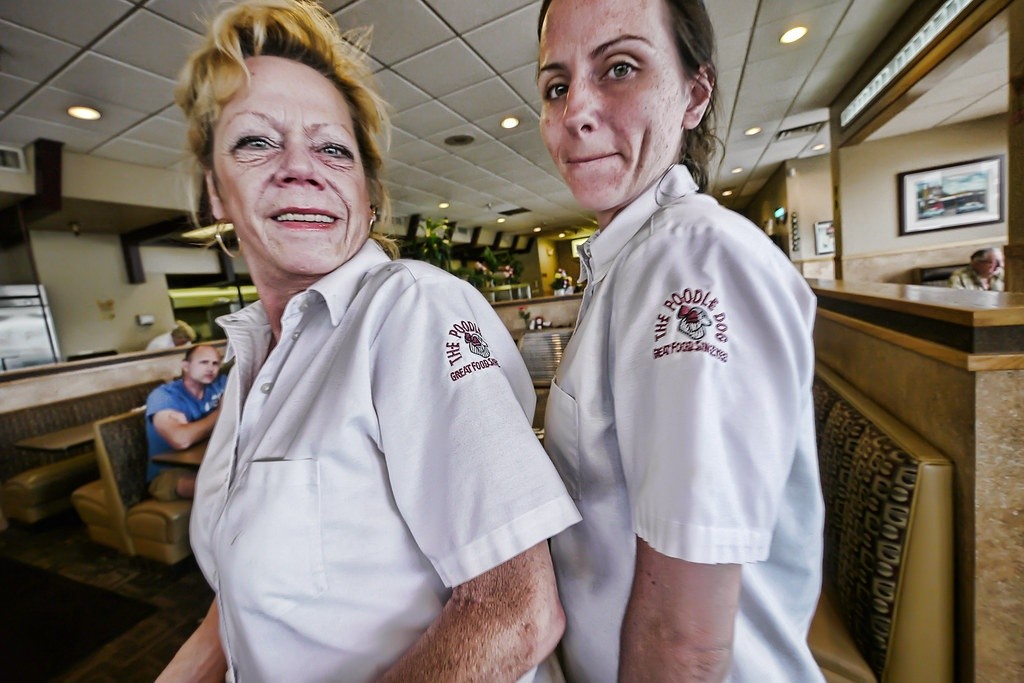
[537,0,824,683]
[148,322,195,346]
[145,345,229,502]
[951,248,1005,294]
[154,0,567,683]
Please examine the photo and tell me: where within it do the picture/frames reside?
[898,153,1006,235]
[814,219,834,256]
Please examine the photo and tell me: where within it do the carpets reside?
[0,550,160,683]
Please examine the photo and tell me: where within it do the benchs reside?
[792,360,955,683]
[72,478,125,554]
[93,404,195,567]
[0,377,167,527]
[912,263,971,288]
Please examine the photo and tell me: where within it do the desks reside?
[151,441,206,470]
[13,420,95,451]
